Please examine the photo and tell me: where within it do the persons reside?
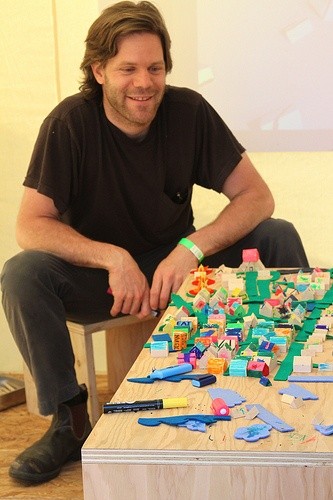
[0,0,309,481]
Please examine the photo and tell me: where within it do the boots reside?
[9,383,93,483]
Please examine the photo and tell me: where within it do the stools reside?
[66,310,167,428]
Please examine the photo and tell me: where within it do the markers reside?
[103,398,188,414]
[147,363,192,379]
[107,287,161,318]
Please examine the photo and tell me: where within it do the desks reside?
[81,268,332,500]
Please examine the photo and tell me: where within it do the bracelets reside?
[177,237,204,263]
